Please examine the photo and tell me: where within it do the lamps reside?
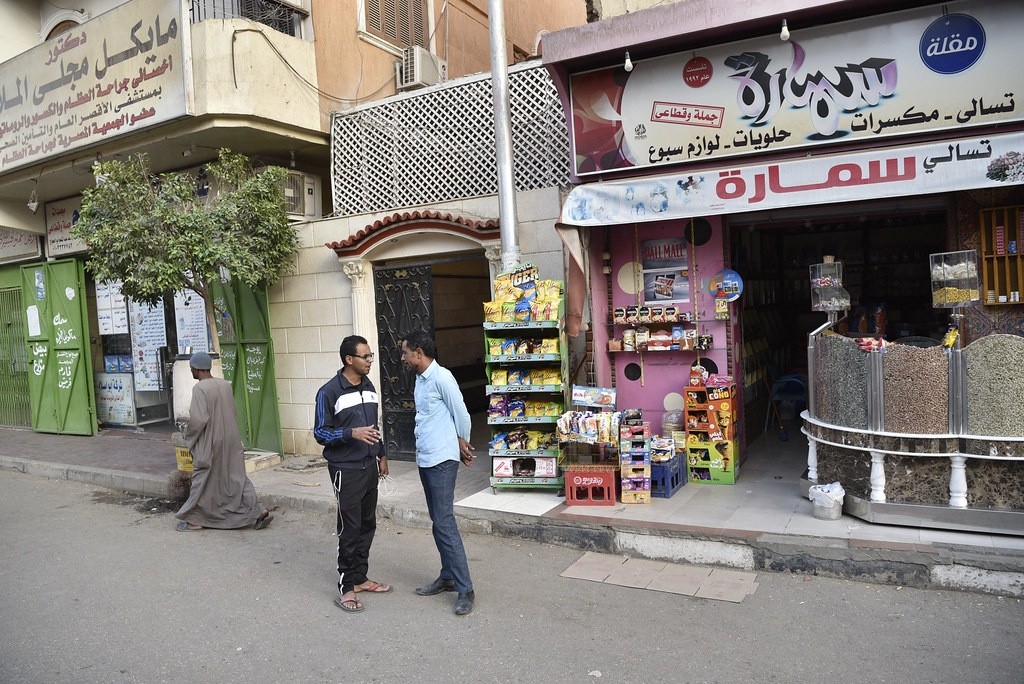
[183,144,197,158]
[24,181,44,215]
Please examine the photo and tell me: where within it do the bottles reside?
[987,290,995,303]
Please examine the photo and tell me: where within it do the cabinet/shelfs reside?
[482,296,567,497]
[729,230,784,443]
[867,215,944,311]
[780,224,866,373]
[603,318,731,350]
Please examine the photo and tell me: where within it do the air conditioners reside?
[400,45,450,88]
[251,165,323,220]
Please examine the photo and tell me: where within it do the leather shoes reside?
[415,577,457,596]
[455,590,475,615]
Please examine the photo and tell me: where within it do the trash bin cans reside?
[808,483,845,521]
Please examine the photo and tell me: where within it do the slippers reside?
[356,582,394,593]
[177,523,203,531]
[255,514,274,530]
[335,592,365,612]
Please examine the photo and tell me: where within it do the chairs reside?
[764,360,809,433]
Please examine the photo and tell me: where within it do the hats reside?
[190,352,212,369]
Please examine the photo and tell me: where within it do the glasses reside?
[351,353,374,363]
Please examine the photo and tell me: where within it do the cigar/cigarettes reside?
[468,455,477,459]
[372,431,376,435]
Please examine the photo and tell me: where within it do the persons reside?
[175,351,274,532]
[314,334,394,612]
[774,277,826,420]
[401,330,475,614]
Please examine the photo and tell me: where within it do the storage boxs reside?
[621,466,651,477]
[651,305,665,322]
[650,331,673,340]
[621,422,652,437]
[634,328,649,351]
[680,337,693,350]
[625,304,639,324]
[671,345,680,351]
[614,307,625,323]
[621,437,652,452]
[638,305,652,323]
[648,340,672,351]
[672,323,683,343]
[681,452,687,485]
[683,382,741,440]
[622,452,650,465]
[621,478,651,490]
[608,338,622,350]
[652,453,681,498]
[621,490,652,504]
[564,469,617,508]
[665,305,680,322]
[688,439,740,484]
